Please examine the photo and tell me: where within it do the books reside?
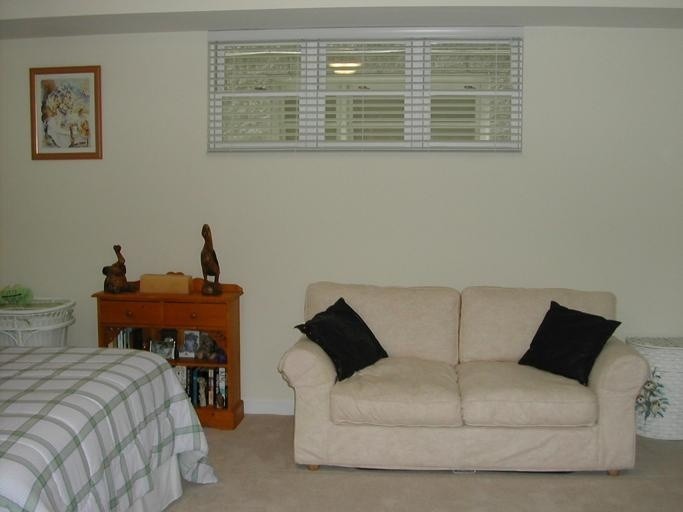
[105,329,228,409]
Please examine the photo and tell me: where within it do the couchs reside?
[277,281,650,474]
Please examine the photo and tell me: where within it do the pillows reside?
[294,298,389,382]
[518,301,621,387]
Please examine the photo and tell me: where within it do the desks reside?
[0,298,76,349]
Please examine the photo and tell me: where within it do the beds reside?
[0,344,217,511]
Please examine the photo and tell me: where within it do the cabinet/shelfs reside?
[93,284,244,430]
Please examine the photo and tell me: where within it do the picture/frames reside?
[29,65,102,160]
